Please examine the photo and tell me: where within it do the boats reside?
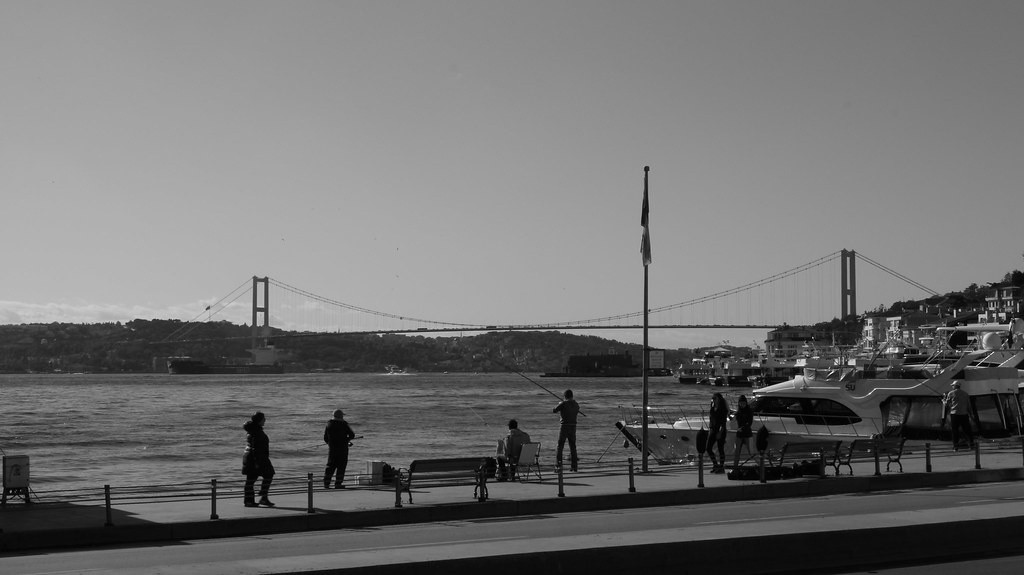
[678,346,733,384]
[746,374,774,388]
[386,365,403,374]
[444,371,449,374]
[166,337,305,375]
[614,317,1024,463]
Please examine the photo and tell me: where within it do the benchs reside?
[836,438,908,476]
[768,439,842,477]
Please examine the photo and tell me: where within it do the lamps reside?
[800,384,807,392]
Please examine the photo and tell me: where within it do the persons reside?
[941,381,974,451]
[706,393,729,475]
[552,390,580,472]
[241,410,275,507]
[733,395,754,466]
[496,420,531,481]
[324,409,355,489]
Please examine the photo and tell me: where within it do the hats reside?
[950,380,961,386]
[738,395,747,402]
[333,409,346,416]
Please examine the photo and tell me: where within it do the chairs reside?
[499,442,542,483]
[392,457,497,506]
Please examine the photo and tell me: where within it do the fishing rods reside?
[491,358,587,418]
[442,380,543,482]
[297,435,365,449]
[900,367,948,400]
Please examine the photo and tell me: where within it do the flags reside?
[640,179,653,265]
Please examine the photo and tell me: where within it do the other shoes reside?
[260,499,275,505]
[715,466,725,473]
[554,469,559,473]
[953,446,958,452]
[570,467,577,472]
[497,477,509,482]
[969,447,975,450]
[335,484,345,489]
[324,477,330,488]
[710,464,718,473]
[245,501,259,507]
